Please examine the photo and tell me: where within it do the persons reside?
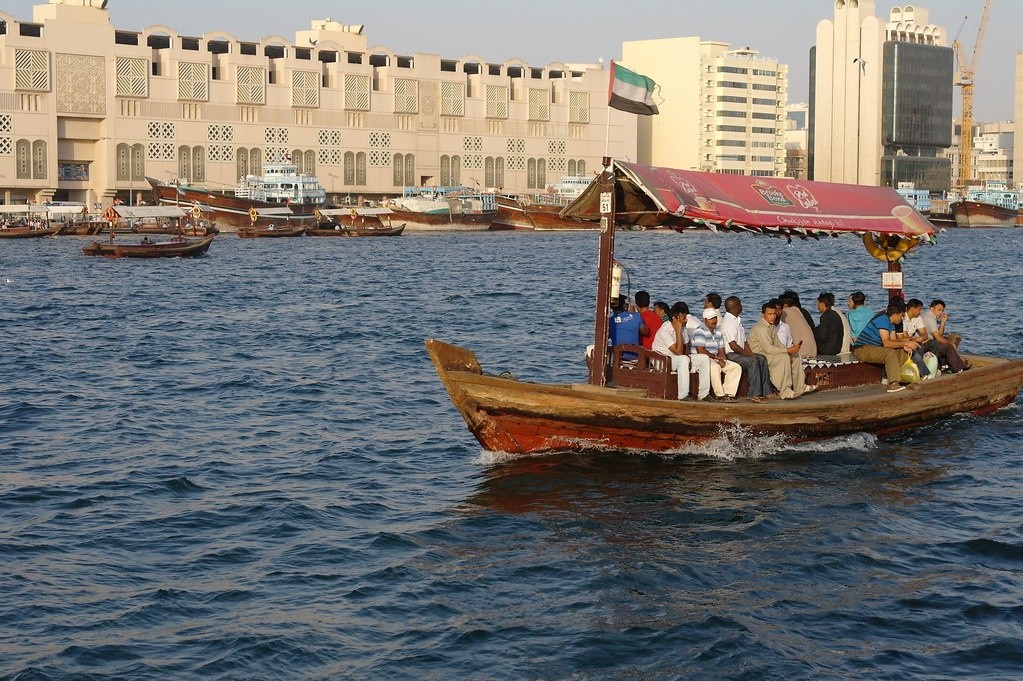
[852,296,968,393]
[689,308,742,403]
[608,291,670,365]
[779,291,815,333]
[720,296,780,403]
[845,292,876,346]
[702,292,724,331]
[768,299,795,349]
[651,301,715,402]
[747,302,819,399]
[814,293,850,355]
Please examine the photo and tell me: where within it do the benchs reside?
[606,344,886,402]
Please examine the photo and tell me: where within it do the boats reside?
[1014,207,1023,227]
[422,162,1023,461]
[142,171,345,232]
[304,208,407,237]
[235,207,306,239]
[950,197,1020,228]
[0,203,66,239]
[51,206,110,236]
[375,184,501,233]
[235,161,327,204]
[81,205,216,258]
[488,172,625,231]
[180,205,220,237]
[895,187,958,228]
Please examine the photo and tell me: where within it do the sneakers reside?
[886,381,905,393]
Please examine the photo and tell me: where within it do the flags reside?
[609,60,660,116]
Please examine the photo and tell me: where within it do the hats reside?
[703,308,719,320]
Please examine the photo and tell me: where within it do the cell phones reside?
[798,340,803,346]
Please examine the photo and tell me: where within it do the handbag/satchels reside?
[923,351,939,377]
[899,349,920,384]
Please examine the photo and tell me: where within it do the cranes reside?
[949,0,991,193]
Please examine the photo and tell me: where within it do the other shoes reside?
[765,394,781,400]
[803,383,819,394]
[784,391,803,400]
[703,394,717,403]
[752,396,768,404]
[681,394,696,402]
[941,363,973,375]
